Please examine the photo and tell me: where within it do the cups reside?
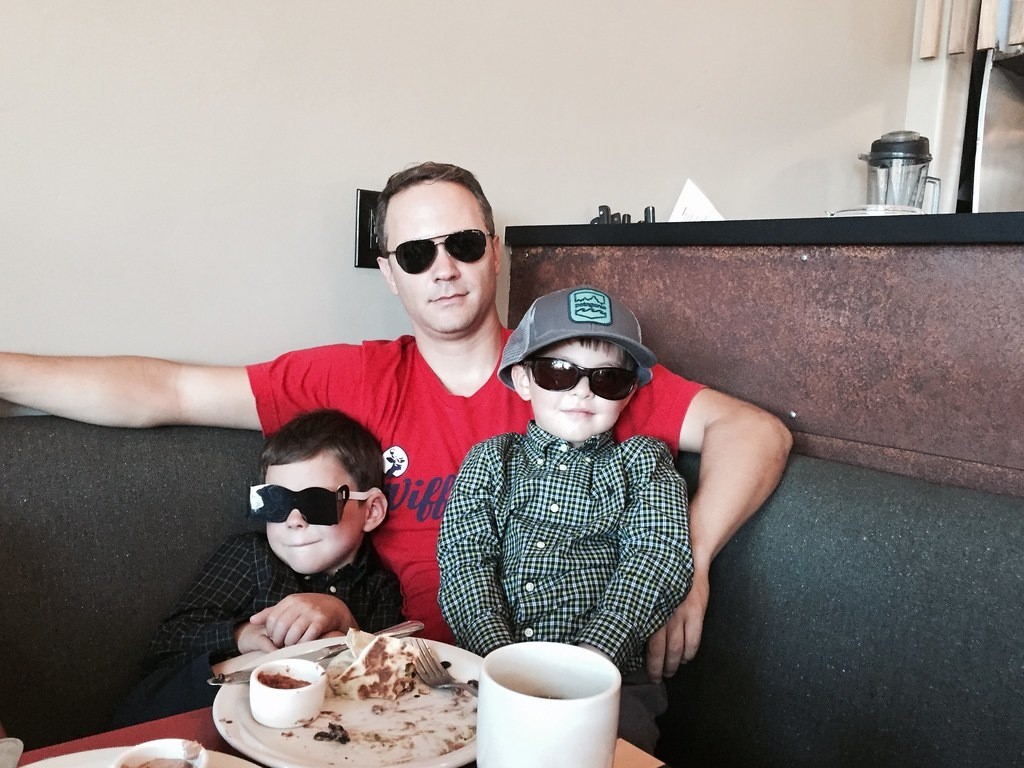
[476,641,621,768]
[249,658,326,728]
[113,738,209,768]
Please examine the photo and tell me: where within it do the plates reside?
[212,636,478,768]
[19,746,264,768]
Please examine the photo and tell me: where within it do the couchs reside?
[0,416,1024,768]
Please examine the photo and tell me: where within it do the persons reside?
[1,159,794,684]
[436,283,695,755]
[132,407,405,719]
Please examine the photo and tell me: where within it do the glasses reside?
[388,229,490,275]
[519,356,637,401]
[249,483,382,527]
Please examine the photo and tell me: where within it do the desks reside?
[15,707,667,768]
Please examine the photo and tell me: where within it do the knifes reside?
[207,620,425,684]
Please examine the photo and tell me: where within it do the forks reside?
[403,637,477,697]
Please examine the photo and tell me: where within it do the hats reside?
[497,284,658,393]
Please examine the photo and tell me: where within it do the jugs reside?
[858,131,940,214]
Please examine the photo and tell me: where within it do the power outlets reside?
[355,189,386,269]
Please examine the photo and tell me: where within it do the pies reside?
[327,626,421,698]
